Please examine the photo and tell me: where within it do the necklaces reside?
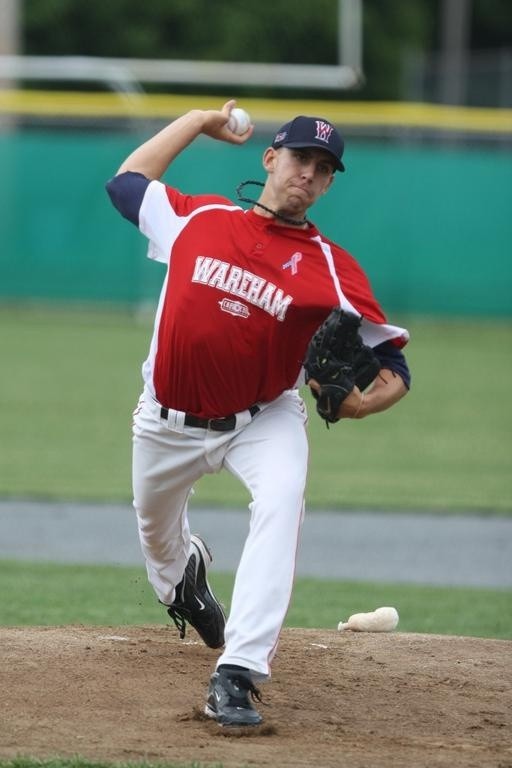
[235,179,310,226]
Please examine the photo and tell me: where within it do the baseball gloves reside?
[305,308,378,429]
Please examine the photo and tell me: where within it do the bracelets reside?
[351,391,366,420]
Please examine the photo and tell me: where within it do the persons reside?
[105,100,414,731]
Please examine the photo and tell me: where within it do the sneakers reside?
[159,533,228,649]
[203,670,261,727]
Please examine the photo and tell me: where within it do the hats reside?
[272,115,345,175]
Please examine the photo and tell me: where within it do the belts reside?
[160,406,261,431]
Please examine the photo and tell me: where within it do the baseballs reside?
[228,108,253,135]
